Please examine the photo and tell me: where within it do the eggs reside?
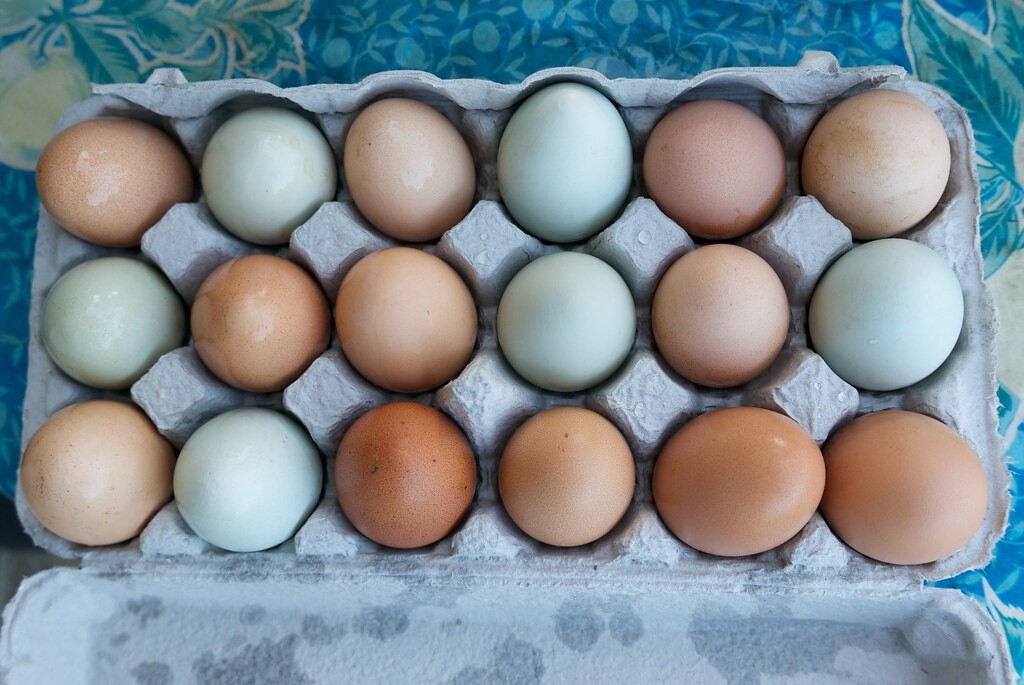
[21,82,989,566]
